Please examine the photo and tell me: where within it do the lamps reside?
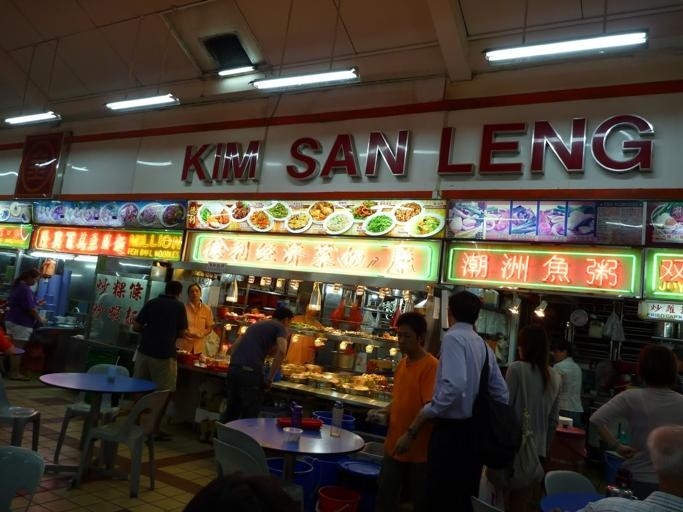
[246,274,433,301]
[2,41,60,126]
[213,65,256,79]
[505,292,551,320]
[483,2,647,67]
[224,271,238,302]
[246,3,363,94]
[101,5,181,113]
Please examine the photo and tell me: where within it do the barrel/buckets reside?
[312,410,357,432]
[36,302,57,327]
[601,452,626,483]
[265,454,384,512]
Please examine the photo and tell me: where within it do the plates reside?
[136,202,164,227]
[1,208,10,221]
[119,202,140,227]
[66,204,90,226]
[196,202,232,230]
[651,205,666,229]
[362,213,396,237]
[263,202,293,221]
[350,204,383,223]
[160,204,183,227]
[307,201,341,225]
[247,209,275,232]
[206,364,227,372]
[322,210,354,236]
[82,205,103,227]
[288,377,369,395]
[284,211,313,234]
[391,200,425,225]
[230,201,254,223]
[49,204,74,225]
[9,201,21,217]
[405,213,445,238]
[99,202,123,227]
[20,208,31,223]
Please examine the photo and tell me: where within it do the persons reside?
[4,266,50,381]
[548,341,584,430]
[589,342,682,496]
[183,467,301,512]
[174,283,215,355]
[0,324,27,356]
[126,280,192,442]
[216,308,296,427]
[416,289,511,511]
[366,313,440,512]
[489,325,555,511]
[285,304,328,363]
[236,300,263,337]
[671,346,682,394]
[577,425,682,511]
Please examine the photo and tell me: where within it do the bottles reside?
[329,400,343,437]
[477,288,497,312]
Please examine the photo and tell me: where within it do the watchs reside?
[406,425,418,440]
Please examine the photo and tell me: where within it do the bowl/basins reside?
[330,350,392,371]
[176,354,199,365]
[53,313,85,328]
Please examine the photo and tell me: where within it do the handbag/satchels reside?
[471,395,522,471]
[507,429,545,492]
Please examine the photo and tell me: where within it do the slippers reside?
[8,375,30,381]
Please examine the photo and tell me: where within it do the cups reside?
[39,310,47,327]
[657,320,682,339]
[290,406,303,427]
[106,365,117,384]
[282,427,303,442]
[192,359,200,367]
